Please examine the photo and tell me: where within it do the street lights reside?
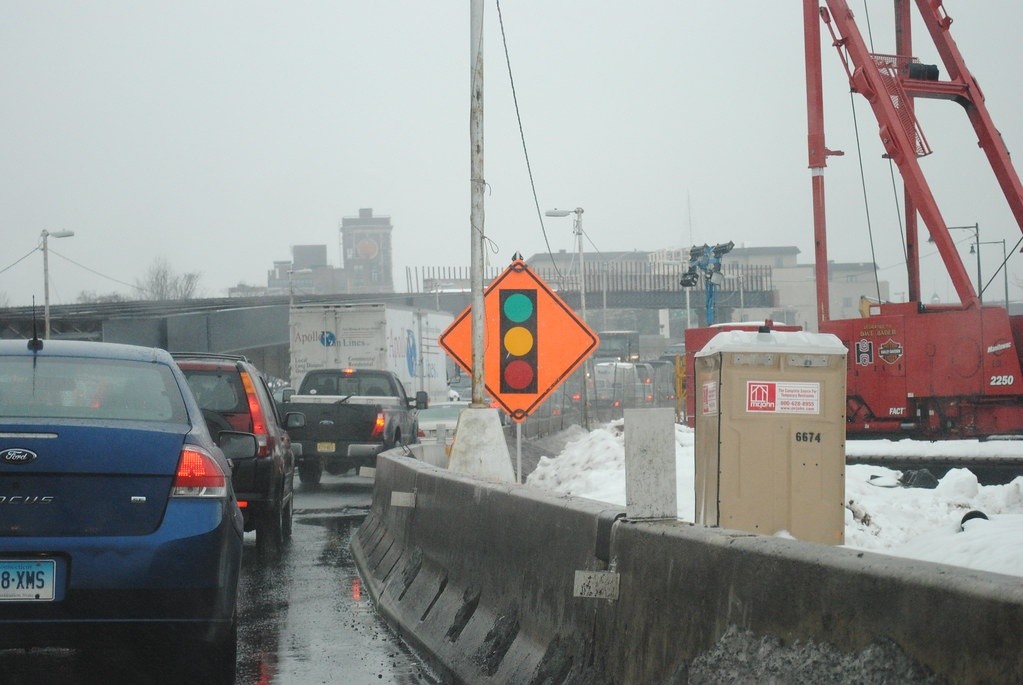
[41,228,75,339]
[542,207,592,433]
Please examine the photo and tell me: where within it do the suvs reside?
[0,336,260,685]
[168,350,309,556]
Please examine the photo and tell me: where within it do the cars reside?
[592,328,687,411]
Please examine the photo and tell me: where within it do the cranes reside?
[677,1,1023,447]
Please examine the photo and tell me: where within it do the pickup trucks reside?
[284,366,430,485]
